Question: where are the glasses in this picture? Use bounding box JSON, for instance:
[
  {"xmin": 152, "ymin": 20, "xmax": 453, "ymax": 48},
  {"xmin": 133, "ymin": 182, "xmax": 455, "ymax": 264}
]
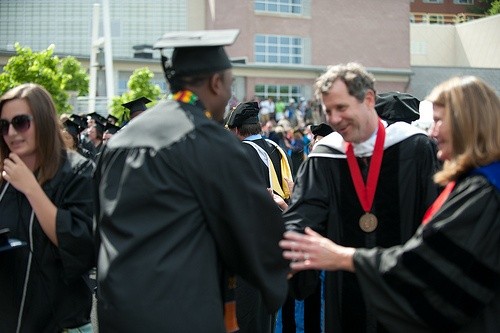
[{"xmin": 0, "ymin": 115, "xmax": 34, "ymax": 136}]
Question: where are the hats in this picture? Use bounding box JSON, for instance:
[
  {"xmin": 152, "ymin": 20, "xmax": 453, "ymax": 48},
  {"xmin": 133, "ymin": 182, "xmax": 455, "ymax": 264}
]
[
  {"xmin": 61, "ymin": 115, "xmax": 88, "ymax": 146},
  {"xmin": 373, "ymin": 91, "xmax": 420, "ymax": 123},
  {"xmin": 227, "ymin": 101, "xmax": 259, "ymax": 126},
  {"xmin": 153, "ymin": 29, "xmax": 241, "ymax": 71},
  {"xmin": 107, "ymin": 115, "xmax": 118, "ymax": 122},
  {"xmin": 95, "ymin": 120, "xmax": 105, "ymax": 132},
  {"xmin": 311, "ymin": 124, "xmax": 334, "ymax": 137},
  {"xmin": 122, "ymin": 97, "xmax": 152, "ymax": 122},
  {"xmin": 88, "ymin": 112, "xmax": 107, "ymax": 123},
  {"xmin": 105, "ymin": 123, "xmax": 120, "ymax": 134}
]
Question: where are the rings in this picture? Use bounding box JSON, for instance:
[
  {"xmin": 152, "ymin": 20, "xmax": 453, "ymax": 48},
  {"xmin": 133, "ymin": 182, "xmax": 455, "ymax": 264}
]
[
  {"xmin": 304, "ymin": 252, "xmax": 310, "ymax": 260},
  {"xmin": 304, "ymin": 260, "xmax": 311, "ymax": 267}
]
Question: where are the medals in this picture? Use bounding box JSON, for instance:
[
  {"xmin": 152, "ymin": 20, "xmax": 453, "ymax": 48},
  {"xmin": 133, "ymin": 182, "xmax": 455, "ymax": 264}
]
[{"xmin": 359, "ymin": 213, "xmax": 378, "ymax": 232}]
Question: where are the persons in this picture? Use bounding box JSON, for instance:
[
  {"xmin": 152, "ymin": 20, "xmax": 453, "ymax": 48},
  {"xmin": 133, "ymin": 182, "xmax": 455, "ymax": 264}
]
[
  {"xmin": 92, "ymin": 29, "xmax": 292, "ymax": 333},
  {"xmin": 0, "ymin": 83, "xmax": 97, "ymax": 333},
  {"xmin": 226, "ymin": 102, "xmax": 295, "ymax": 333},
  {"xmin": 60, "ymin": 91, "xmax": 420, "ymax": 182},
  {"xmin": 280, "ymin": 73, "xmax": 500, "ymax": 333},
  {"xmin": 282, "ymin": 61, "xmax": 444, "ymax": 333}
]
